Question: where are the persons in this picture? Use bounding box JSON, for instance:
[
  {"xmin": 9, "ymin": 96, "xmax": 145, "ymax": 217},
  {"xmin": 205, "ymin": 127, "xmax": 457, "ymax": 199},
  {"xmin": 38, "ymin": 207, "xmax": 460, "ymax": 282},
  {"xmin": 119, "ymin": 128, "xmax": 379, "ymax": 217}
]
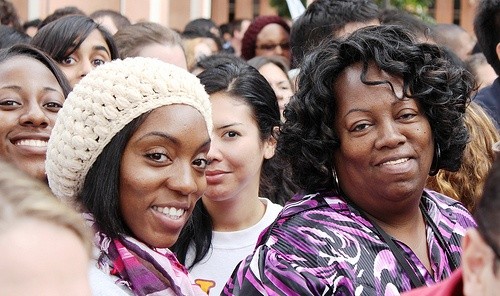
[
  {"xmin": 292, "ymin": 0, "xmax": 440, "ymax": 60},
  {"xmin": 180, "ymin": 16, "xmax": 300, "ymax": 128},
  {"xmin": 32, "ymin": 16, "xmax": 119, "ymax": 91},
  {"xmin": 221, "ymin": 25, "xmax": 479, "ymax": 296},
  {"xmin": 0, "ymin": 43, "xmax": 70, "ymax": 181},
  {"xmin": 0, "ymin": 0, "xmax": 131, "ymax": 42},
  {"xmin": 44, "ymin": 56, "xmax": 214, "ymax": 296},
  {"xmin": 111, "ymin": 21, "xmax": 188, "ymax": 72},
  {"xmin": 463, "ymin": 0, "xmax": 500, "ymax": 133},
  {"xmin": 178, "ymin": 62, "xmax": 284, "ymax": 296},
  {"xmin": 426, "ymin": 95, "xmax": 500, "ymax": 215},
  {"xmin": 462, "ymin": 153, "xmax": 500, "ymax": 296},
  {"xmin": 0, "ymin": 160, "xmax": 95, "ymax": 296}
]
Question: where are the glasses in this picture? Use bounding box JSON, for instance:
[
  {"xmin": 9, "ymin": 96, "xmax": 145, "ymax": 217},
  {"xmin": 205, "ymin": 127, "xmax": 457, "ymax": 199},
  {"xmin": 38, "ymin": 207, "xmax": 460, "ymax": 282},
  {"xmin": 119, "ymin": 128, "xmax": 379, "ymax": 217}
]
[{"xmin": 254, "ymin": 42, "xmax": 290, "ymax": 50}]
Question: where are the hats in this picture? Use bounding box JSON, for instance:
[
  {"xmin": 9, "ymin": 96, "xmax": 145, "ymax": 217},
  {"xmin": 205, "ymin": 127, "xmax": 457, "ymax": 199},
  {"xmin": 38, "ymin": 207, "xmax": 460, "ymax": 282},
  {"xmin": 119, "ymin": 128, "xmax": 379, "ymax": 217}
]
[
  {"xmin": 43, "ymin": 55, "xmax": 212, "ymax": 205},
  {"xmin": 183, "ymin": 18, "xmax": 223, "ymax": 49},
  {"xmin": 241, "ymin": 15, "xmax": 291, "ymax": 61}
]
